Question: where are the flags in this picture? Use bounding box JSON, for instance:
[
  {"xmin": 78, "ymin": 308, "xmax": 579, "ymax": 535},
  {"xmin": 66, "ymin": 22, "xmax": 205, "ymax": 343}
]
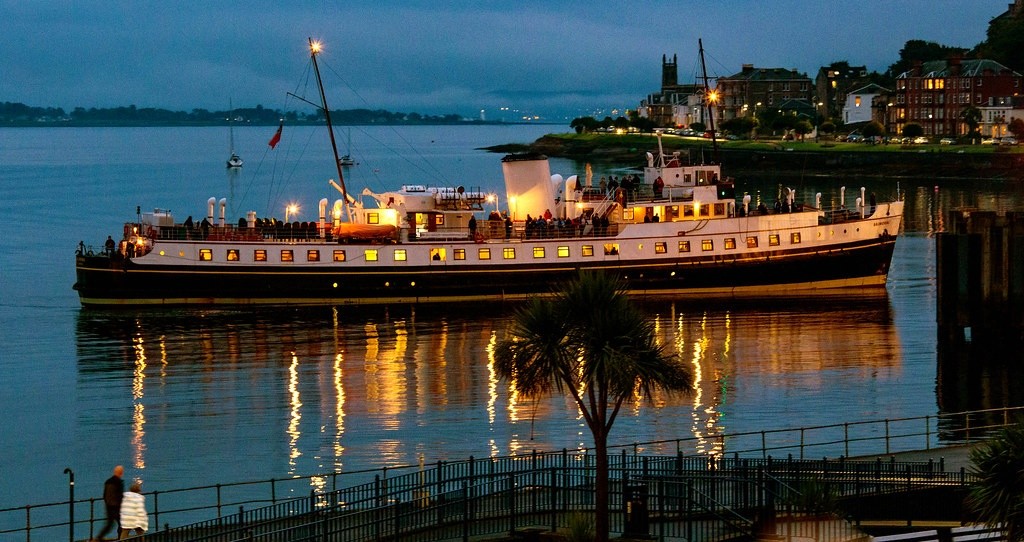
[{"xmin": 268, "ymin": 122, "xmax": 282, "ymax": 149}]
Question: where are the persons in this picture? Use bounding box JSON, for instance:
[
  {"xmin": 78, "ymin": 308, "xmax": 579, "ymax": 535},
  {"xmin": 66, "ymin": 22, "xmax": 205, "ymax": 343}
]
[
  {"xmin": 184, "ymin": 216, "xmax": 194, "ymax": 240},
  {"xmin": 469, "ymin": 174, "xmax": 797, "ymax": 239},
  {"xmin": 94, "ymin": 466, "xmax": 148, "ymax": 542},
  {"xmin": 201, "ymin": 218, "xmax": 214, "ymax": 241},
  {"xmin": 105, "ymin": 236, "xmax": 115, "ymax": 256}
]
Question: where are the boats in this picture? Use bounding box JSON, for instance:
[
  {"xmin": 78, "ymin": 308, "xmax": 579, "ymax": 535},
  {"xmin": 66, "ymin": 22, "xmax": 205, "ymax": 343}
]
[
  {"xmin": 67, "ymin": 35, "xmax": 906, "ymax": 313},
  {"xmin": 226, "ymin": 153, "xmax": 245, "ymax": 167},
  {"xmin": 337, "ymin": 155, "xmax": 355, "ymax": 167}
]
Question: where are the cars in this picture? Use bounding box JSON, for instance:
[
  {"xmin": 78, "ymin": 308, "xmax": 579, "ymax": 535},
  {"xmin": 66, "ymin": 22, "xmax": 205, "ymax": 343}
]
[
  {"xmin": 914, "ymin": 136, "xmax": 929, "ymax": 144},
  {"xmin": 835, "ymin": 130, "xmax": 915, "ymax": 144},
  {"xmin": 940, "ymin": 138, "xmax": 957, "ymax": 145},
  {"xmin": 653, "ymin": 127, "xmax": 741, "ymax": 141},
  {"xmin": 782, "ymin": 134, "xmax": 793, "ymax": 140},
  {"xmin": 982, "ymin": 138, "xmax": 1000, "ymax": 145},
  {"xmin": 1001, "ymin": 137, "xmax": 1018, "ymax": 145},
  {"xmin": 606, "ymin": 125, "xmax": 638, "ymax": 134}
]
